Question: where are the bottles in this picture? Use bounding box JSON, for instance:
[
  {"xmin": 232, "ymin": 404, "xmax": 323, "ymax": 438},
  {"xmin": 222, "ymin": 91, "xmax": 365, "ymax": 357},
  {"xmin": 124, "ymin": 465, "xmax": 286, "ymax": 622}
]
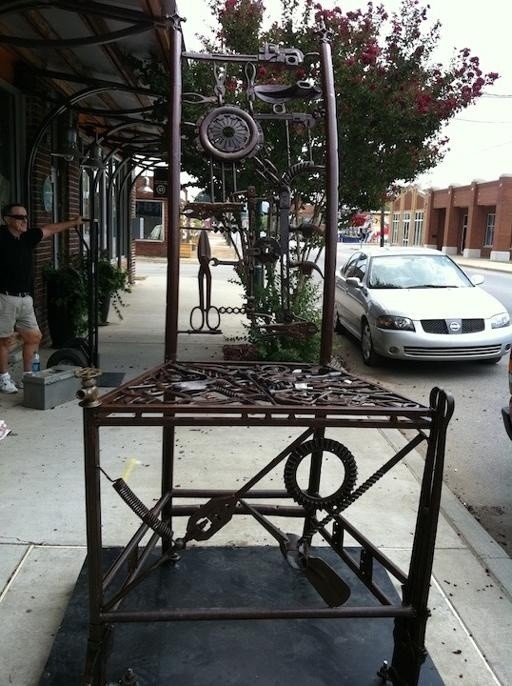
[{"xmin": 31, "ymin": 350, "xmax": 41, "ymax": 377}]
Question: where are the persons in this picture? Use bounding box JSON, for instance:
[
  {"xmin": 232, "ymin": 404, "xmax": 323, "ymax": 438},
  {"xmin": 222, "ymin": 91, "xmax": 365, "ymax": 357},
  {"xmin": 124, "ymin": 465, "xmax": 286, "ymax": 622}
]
[
  {"xmin": 356, "ymin": 228, "xmax": 365, "ymax": 248},
  {"xmin": 0, "ymin": 204, "xmax": 86, "ymax": 393}
]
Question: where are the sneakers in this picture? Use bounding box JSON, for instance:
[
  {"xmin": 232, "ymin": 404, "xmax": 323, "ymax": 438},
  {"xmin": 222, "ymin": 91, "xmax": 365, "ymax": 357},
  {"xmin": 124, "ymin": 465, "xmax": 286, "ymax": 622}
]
[{"xmin": 0, "ymin": 376, "xmax": 18, "ymax": 394}]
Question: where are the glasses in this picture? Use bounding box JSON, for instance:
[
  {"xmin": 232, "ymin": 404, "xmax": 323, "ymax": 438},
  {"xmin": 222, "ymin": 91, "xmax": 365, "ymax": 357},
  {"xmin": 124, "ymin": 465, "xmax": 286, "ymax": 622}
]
[{"xmin": 7, "ymin": 214, "xmax": 27, "ymax": 219}]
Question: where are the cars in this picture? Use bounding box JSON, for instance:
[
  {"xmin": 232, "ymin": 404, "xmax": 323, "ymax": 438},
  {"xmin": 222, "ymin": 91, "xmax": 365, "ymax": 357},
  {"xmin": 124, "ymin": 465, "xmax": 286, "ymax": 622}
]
[{"xmin": 334, "ymin": 246, "xmax": 511, "ymax": 367}]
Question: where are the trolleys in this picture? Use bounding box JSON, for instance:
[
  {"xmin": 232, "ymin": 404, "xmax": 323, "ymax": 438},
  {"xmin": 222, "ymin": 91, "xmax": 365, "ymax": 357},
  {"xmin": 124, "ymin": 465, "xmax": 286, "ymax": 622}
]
[{"xmin": 46, "ymin": 219, "xmax": 126, "ymax": 387}]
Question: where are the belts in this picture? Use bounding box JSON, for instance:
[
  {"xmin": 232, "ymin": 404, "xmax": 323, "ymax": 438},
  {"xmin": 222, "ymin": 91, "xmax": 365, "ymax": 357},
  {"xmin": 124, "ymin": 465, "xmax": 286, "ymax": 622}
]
[{"xmin": 1, "ymin": 290, "xmax": 30, "ymax": 297}]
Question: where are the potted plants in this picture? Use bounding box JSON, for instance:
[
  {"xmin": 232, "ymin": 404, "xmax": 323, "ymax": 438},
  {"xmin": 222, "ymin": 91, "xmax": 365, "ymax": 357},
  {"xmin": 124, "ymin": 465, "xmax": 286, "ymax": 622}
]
[{"xmin": 41, "ymin": 246, "xmax": 137, "ymax": 349}]
